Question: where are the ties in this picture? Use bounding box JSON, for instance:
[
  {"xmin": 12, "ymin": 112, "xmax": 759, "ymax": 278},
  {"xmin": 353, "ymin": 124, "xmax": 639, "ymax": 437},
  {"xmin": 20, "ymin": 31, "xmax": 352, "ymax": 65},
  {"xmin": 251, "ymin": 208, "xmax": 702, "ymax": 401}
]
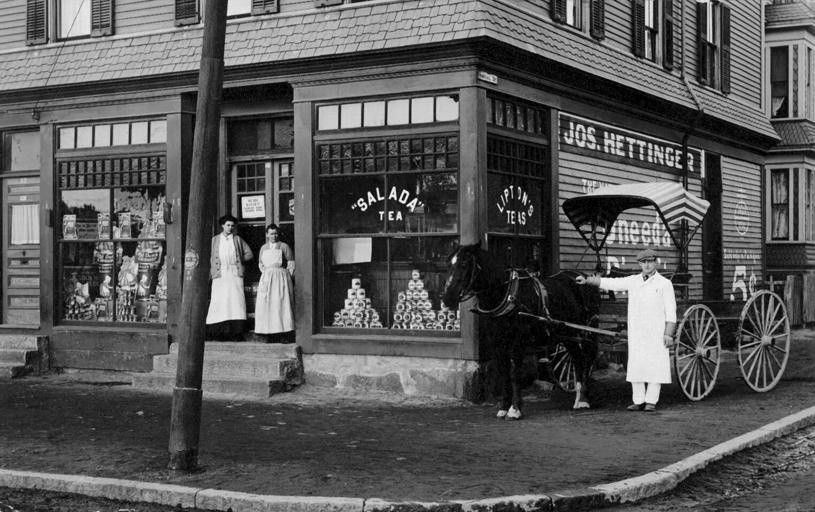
[{"xmin": 643, "ymin": 274, "xmax": 649, "ymax": 281}]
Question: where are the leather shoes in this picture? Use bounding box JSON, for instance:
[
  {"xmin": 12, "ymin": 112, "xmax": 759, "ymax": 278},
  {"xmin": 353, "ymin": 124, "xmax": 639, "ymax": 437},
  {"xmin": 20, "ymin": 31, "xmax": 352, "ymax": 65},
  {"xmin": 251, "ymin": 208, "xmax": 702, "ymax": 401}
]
[
  {"xmin": 627, "ymin": 404, "xmax": 644, "ymax": 411},
  {"xmin": 644, "ymin": 403, "xmax": 656, "ymax": 411}
]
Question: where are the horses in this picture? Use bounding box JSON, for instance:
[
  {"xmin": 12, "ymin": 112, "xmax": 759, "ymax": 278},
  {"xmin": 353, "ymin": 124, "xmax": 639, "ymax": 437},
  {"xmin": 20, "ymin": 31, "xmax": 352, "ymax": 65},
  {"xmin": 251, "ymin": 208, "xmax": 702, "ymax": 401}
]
[{"xmin": 438, "ymin": 238, "xmax": 602, "ymax": 422}]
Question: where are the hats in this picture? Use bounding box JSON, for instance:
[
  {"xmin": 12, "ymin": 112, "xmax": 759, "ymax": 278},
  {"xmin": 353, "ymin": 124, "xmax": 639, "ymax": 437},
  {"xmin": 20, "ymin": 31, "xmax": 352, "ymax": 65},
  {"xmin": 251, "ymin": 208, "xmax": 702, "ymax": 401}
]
[{"xmin": 636, "ymin": 250, "xmax": 657, "ymax": 263}]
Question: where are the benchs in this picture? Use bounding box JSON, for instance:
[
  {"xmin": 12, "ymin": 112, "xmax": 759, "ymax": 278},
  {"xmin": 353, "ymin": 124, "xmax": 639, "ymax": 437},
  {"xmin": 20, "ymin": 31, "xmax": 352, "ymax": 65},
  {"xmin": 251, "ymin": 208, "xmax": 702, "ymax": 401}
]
[{"xmin": 603, "ymin": 267, "xmax": 693, "ymax": 299}]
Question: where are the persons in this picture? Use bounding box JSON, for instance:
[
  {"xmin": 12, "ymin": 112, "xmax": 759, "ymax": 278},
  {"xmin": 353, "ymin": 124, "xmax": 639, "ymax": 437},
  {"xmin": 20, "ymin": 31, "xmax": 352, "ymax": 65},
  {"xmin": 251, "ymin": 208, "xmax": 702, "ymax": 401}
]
[
  {"xmin": 206, "ymin": 212, "xmax": 254, "ymax": 343},
  {"xmin": 574, "ymin": 248, "xmax": 677, "ymax": 412},
  {"xmin": 255, "ymin": 223, "xmax": 295, "ymax": 344}
]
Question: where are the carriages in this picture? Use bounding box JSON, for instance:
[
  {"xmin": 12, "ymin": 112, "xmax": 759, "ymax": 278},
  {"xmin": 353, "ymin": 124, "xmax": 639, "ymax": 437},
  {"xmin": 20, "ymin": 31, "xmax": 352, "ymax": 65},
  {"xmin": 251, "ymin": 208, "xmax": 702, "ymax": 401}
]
[{"xmin": 439, "ymin": 181, "xmax": 790, "ymax": 420}]
